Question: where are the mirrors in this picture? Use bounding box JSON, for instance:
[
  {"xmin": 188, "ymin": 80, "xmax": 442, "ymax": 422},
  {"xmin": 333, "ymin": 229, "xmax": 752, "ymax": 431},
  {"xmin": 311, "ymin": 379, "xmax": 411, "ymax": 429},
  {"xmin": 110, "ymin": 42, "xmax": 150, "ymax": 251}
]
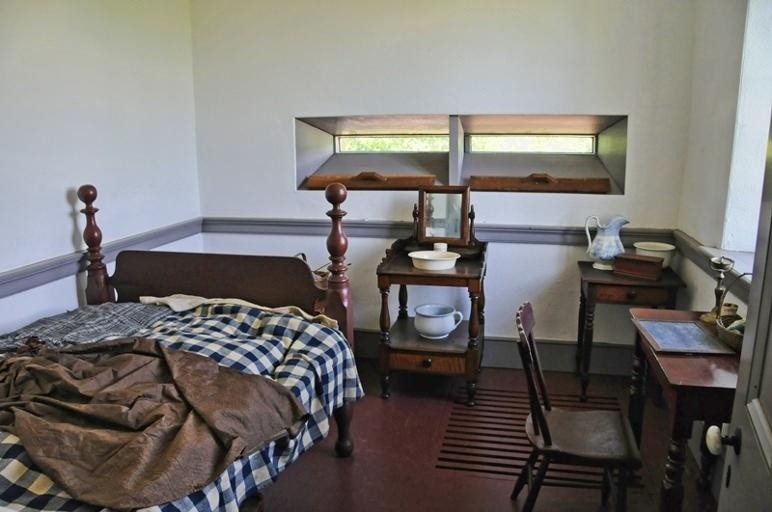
[{"xmin": 412, "ymin": 185, "xmax": 484, "ymax": 248}]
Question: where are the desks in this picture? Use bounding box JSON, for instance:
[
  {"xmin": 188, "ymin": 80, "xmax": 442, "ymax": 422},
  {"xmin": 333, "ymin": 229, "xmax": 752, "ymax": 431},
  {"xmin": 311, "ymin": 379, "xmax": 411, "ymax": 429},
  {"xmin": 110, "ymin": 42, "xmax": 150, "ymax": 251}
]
[
  {"xmin": 628, "ymin": 310, "xmax": 741, "ymax": 512},
  {"xmin": 577, "ymin": 262, "xmax": 685, "ymax": 404},
  {"xmin": 377, "ymin": 253, "xmax": 487, "ymax": 407}
]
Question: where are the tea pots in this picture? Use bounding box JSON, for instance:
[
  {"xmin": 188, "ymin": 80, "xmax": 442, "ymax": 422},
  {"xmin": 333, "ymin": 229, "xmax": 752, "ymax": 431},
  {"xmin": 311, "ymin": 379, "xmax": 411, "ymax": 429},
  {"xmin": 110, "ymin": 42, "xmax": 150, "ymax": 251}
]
[{"xmin": 585, "ymin": 217, "xmax": 630, "ymax": 271}]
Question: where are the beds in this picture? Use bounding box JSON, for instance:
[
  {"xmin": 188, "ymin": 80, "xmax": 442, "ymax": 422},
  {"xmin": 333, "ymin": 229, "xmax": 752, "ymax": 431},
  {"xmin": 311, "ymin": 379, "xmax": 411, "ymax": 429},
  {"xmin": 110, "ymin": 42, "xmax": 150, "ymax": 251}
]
[{"xmin": 0, "ymin": 183, "xmax": 366, "ymax": 511}]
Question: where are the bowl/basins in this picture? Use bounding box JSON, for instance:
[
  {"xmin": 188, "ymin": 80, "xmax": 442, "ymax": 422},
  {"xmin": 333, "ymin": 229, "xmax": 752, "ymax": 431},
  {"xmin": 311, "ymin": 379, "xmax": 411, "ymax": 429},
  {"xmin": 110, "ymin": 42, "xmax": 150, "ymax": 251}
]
[
  {"xmin": 634, "ymin": 239, "xmax": 677, "ymax": 268},
  {"xmin": 406, "ymin": 250, "xmax": 460, "ymax": 270}
]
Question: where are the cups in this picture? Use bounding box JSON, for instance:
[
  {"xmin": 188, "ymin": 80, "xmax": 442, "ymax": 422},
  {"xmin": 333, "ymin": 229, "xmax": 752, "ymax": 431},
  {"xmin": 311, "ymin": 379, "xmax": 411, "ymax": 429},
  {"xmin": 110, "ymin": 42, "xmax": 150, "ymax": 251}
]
[{"xmin": 413, "ymin": 303, "xmax": 463, "ymax": 341}]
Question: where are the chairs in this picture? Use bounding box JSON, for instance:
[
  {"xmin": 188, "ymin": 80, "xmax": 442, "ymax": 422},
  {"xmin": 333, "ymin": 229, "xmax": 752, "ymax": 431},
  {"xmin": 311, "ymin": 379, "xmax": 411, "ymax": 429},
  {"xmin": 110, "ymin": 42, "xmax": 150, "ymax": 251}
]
[{"xmin": 510, "ymin": 303, "xmax": 643, "ymax": 512}]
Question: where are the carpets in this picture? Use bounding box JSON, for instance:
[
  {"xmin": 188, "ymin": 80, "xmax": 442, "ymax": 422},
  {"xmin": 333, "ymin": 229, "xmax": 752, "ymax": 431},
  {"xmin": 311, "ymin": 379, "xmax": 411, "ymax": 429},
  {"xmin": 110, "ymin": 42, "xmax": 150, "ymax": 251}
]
[{"xmin": 434, "ymin": 383, "xmax": 652, "ymax": 490}]
